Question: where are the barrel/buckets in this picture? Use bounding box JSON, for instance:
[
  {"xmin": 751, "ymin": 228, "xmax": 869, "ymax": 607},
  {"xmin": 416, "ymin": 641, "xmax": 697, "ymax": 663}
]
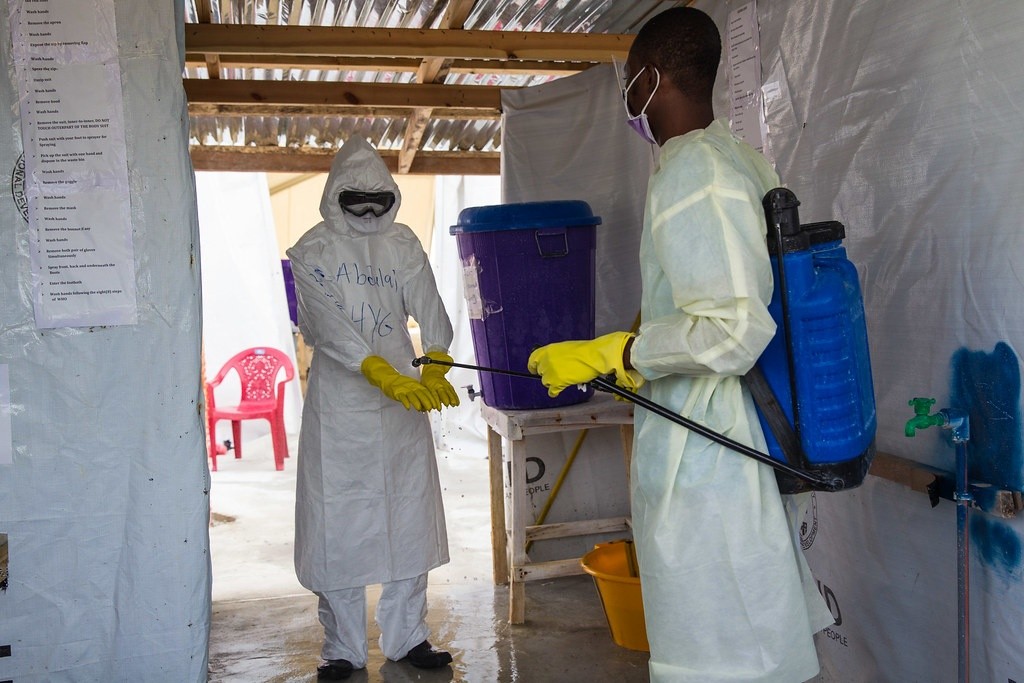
[
  {"xmin": 580, "ymin": 540, "xmax": 651, "ymax": 651},
  {"xmin": 750, "ymin": 187, "xmax": 878, "ymax": 493},
  {"xmin": 450, "ymin": 199, "xmax": 602, "ymax": 410}
]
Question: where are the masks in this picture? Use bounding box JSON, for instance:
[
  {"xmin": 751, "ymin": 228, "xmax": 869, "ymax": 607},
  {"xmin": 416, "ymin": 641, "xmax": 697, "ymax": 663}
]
[
  {"xmin": 623, "ymin": 66, "xmax": 660, "ymax": 144},
  {"xmin": 342, "ymin": 203, "xmax": 386, "ymax": 233}
]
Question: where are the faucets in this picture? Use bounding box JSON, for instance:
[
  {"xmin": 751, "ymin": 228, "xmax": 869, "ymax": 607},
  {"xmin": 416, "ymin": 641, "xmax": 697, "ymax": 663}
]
[{"xmin": 902, "ymin": 396, "xmax": 945, "ymax": 439}]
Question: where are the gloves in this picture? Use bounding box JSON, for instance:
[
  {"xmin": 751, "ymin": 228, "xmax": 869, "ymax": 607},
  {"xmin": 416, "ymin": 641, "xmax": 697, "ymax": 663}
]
[
  {"xmin": 528, "ymin": 331, "xmax": 645, "ymax": 398},
  {"xmin": 613, "ymin": 380, "xmax": 641, "ymax": 405},
  {"xmin": 361, "ymin": 356, "xmax": 437, "ymax": 412},
  {"xmin": 421, "ymin": 351, "xmax": 460, "ymax": 407}
]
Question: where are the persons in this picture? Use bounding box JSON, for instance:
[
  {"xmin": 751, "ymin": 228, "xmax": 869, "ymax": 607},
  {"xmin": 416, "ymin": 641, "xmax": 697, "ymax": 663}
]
[
  {"xmin": 287, "ymin": 133, "xmax": 462, "ymax": 683},
  {"xmin": 526, "ymin": 6, "xmax": 835, "ymax": 683}
]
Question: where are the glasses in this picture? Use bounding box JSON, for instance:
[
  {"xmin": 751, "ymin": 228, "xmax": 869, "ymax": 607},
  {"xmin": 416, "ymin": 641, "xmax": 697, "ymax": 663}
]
[{"xmin": 338, "ymin": 191, "xmax": 396, "ymax": 217}]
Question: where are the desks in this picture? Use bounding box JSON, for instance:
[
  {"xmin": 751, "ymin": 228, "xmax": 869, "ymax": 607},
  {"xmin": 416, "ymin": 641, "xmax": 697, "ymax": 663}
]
[{"xmin": 481, "ymin": 390, "xmax": 635, "ymax": 626}]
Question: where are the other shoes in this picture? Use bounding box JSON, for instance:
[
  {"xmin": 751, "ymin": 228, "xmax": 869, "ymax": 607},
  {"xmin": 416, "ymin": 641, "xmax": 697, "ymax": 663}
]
[
  {"xmin": 316, "ymin": 659, "xmax": 352, "ymax": 680},
  {"xmin": 387, "ymin": 639, "xmax": 453, "ymax": 668}
]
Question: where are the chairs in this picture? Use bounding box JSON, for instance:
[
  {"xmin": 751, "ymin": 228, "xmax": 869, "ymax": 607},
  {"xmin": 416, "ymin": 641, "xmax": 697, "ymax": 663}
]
[{"xmin": 205, "ymin": 347, "xmax": 295, "ymax": 470}]
